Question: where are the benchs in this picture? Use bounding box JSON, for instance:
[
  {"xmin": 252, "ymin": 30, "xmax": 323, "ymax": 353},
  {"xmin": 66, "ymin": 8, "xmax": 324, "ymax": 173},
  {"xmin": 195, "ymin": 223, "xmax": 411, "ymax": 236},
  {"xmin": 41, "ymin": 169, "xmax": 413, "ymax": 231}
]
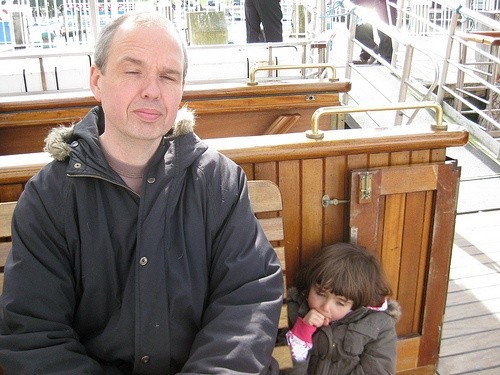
[{"xmin": 0, "ymin": 180, "xmax": 294, "ymax": 375}]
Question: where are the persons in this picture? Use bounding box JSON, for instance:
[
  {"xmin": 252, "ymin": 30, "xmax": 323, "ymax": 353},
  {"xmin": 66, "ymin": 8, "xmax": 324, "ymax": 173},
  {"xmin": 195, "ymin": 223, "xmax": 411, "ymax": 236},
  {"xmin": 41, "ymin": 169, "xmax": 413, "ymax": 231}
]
[
  {"xmin": 271, "ymin": 240, "xmax": 402, "ymax": 375},
  {"xmin": 244, "ymin": 0, "xmax": 283, "ymax": 42},
  {"xmin": 345, "ymin": 11, "xmax": 393, "ymax": 65},
  {"xmin": 0, "ymin": 10, "xmax": 285, "ymax": 375}
]
